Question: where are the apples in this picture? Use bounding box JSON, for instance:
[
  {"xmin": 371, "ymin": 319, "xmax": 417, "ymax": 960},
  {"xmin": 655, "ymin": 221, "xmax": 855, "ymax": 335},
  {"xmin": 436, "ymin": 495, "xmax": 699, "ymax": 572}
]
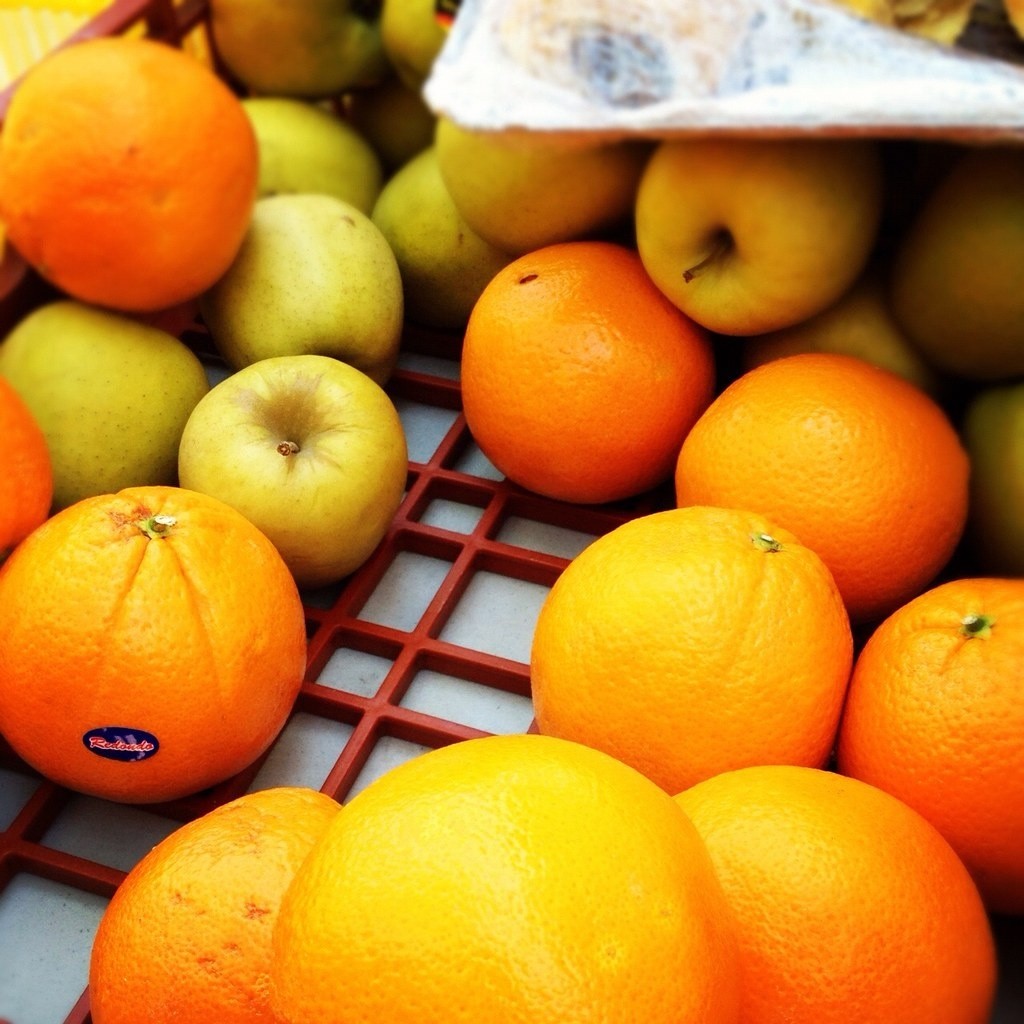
[{"xmin": 0, "ymin": 0, "xmax": 1024, "ymax": 594}]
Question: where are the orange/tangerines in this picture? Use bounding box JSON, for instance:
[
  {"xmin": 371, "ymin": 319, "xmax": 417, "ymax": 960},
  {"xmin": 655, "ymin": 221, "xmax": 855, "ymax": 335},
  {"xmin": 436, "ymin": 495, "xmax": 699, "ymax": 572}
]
[{"xmin": 0, "ymin": 36, "xmax": 1024, "ymax": 1024}]
